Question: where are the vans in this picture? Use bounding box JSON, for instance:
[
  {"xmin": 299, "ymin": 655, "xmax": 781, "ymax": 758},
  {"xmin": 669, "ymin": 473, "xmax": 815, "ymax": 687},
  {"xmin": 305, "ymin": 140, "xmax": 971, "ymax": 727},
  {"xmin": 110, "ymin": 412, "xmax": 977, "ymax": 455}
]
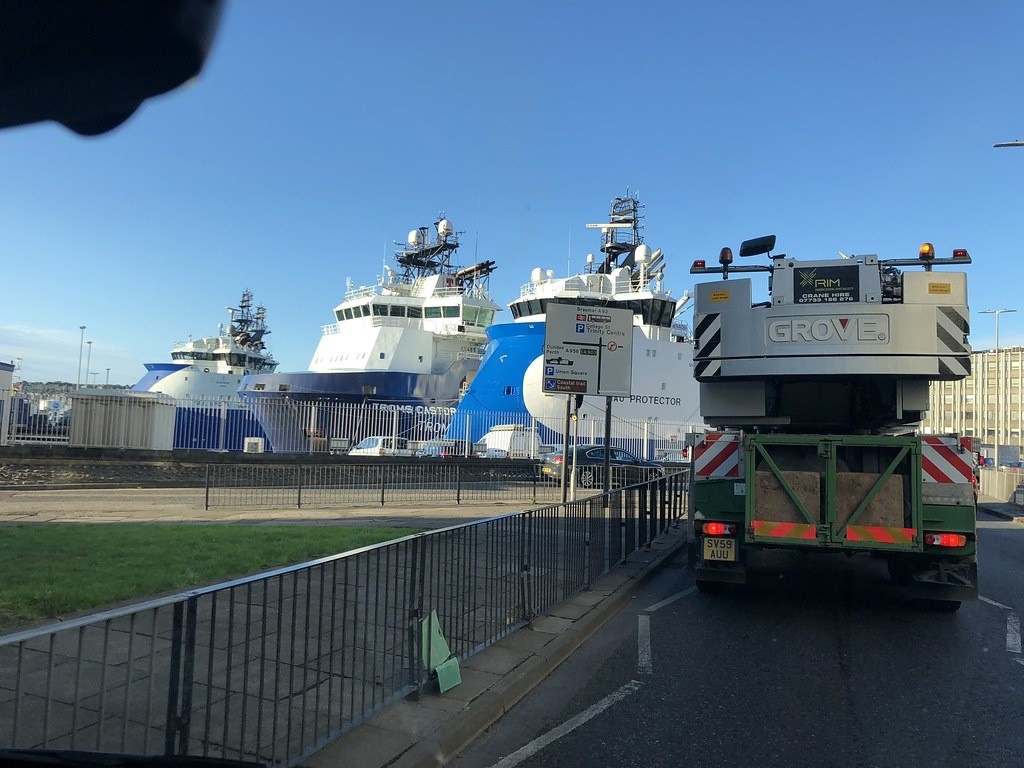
[
  {"xmin": 347, "ymin": 435, "xmax": 410, "ymax": 456},
  {"xmin": 475, "ymin": 425, "xmax": 545, "ymax": 460}
]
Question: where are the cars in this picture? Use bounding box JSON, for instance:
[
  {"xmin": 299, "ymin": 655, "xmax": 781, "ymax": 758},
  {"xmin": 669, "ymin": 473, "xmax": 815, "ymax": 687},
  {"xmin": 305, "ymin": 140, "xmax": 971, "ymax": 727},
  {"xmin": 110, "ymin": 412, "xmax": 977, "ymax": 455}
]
[
  {"xmin": 544, "ymin": 445, "xmax": 665, "ymax": 489},
  {"xmin": 979, "ymin": 455, "xmax": 1024, "ymax": 472}
]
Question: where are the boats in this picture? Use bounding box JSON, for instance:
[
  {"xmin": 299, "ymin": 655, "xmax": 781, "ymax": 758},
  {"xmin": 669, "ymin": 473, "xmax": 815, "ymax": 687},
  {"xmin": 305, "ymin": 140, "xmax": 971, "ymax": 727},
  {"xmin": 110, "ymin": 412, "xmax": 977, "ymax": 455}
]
[
  {"xmin": 124, "ymin": 287, "xmax": 277, "ymax": 452},
  {"xmin": 425, "ymin": 182, "xmax": 716, "ymax": 464},
  {"xmin": 236, "ymin": 207, "xmax": 505, "ymax": 453}
]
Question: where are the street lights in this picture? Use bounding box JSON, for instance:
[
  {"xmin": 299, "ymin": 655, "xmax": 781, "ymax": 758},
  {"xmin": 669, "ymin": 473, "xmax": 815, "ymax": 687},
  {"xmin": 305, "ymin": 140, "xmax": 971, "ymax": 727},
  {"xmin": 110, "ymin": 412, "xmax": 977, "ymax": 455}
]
[
  {"xmin": 106, "ymin": 368, "xmax": 112, "ymax": 385},
  {"xmin": 91, "ymin": 372, "xmax": 99, "ymax": 386},
  {"xmin": 979, "ymin": 308, "xmax": 1018, "ymax": 470},
  {"xmin": 85, "ymin": 340, "xmax": 93, "ymax": 385},
  {"xmin": 76, "ymin": 326, "xmax": 87, "ymax": 389}
]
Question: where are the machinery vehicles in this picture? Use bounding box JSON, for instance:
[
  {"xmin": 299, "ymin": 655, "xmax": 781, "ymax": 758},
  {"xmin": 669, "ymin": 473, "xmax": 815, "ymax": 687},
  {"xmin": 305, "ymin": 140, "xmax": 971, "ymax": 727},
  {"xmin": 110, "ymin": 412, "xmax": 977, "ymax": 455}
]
[{"xmin": 690, "ymin": 235, "xmax": 981, "ymax": 608}]
